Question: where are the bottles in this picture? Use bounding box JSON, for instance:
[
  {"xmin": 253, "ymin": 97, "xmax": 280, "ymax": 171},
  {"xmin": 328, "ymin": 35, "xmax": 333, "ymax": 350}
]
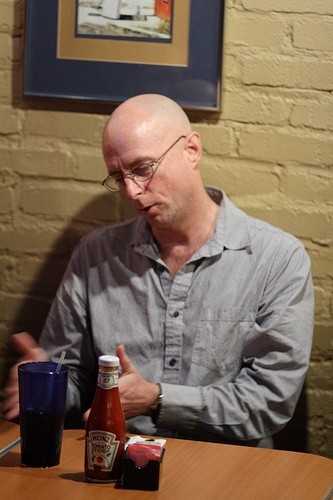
[{"xmin": 83, "ymin": 353, "xmax": 125, "ymax": 485}]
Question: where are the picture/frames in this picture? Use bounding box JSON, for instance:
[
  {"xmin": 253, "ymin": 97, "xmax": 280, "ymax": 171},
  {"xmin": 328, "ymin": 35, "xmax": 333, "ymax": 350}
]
[{"xmin": 23, "ymin": 0, "xmax": 225, "ymax": 114}]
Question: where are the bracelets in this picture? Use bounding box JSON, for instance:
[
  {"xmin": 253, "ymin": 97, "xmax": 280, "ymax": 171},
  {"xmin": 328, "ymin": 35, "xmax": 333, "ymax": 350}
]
[{"xmin": 150, "ymin": 394, "xmax": 163, "ymax": 425}]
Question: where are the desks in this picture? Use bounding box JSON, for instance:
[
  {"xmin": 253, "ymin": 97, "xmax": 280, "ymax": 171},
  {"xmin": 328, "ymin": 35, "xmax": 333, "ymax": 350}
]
[{"xmin": 0, "ymin": 421, "xmax": 333, "ymax": 500}]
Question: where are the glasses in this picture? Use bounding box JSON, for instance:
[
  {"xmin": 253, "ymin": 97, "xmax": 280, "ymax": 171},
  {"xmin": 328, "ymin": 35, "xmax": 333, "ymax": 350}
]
[{"xmin": 102, "ymin": 136, "xmax": 187, "ymax": 193}]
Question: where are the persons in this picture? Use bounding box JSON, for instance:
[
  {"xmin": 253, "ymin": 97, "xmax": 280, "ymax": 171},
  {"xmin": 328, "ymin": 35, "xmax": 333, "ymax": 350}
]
[{"xmin": 3, "ymin": 94, "xmax": 315, "ymax": 448}]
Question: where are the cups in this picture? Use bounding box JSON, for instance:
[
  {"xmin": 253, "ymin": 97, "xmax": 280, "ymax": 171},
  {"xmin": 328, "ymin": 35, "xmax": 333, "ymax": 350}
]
[{"xmin": 17, "ymin": 358, "xmax": 69, "ymax": 469}]
